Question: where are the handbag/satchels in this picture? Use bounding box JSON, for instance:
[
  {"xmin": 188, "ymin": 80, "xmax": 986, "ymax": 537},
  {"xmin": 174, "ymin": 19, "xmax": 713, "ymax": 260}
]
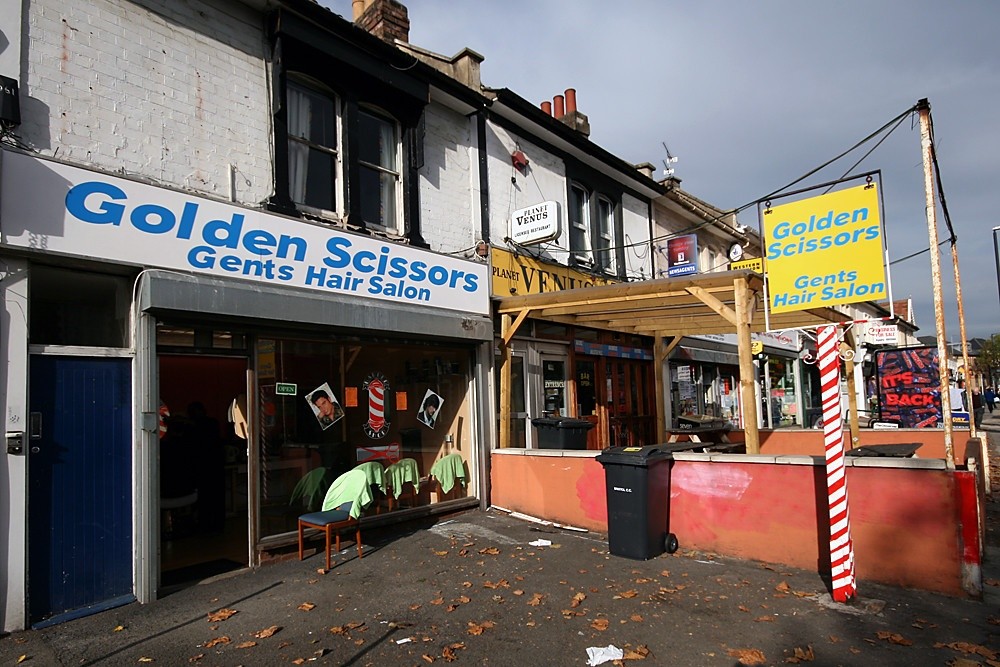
[{"xmin": 993, "ymin": 395, "xmax": 1000, "ymax": 402}]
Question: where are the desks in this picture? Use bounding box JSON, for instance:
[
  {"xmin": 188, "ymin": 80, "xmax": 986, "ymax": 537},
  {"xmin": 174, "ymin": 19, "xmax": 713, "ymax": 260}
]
[{"xmin": 654, "ymin": 427, "xmax": 731, "ymax": 454}]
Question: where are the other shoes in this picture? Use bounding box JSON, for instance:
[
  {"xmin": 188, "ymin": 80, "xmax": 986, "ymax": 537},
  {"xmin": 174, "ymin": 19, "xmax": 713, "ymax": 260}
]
[{"xmin": 977, "ymin": 426, "xmax": 981, "ymax": 430}]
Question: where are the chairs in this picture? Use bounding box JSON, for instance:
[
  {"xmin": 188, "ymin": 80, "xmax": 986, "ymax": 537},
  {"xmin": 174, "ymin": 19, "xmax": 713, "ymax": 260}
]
[
  {"xmin": 298, "ymin": 455, "xmax": 462, "ymax": 571},
  {"xmin": 289, "ymin": 467, "xmax": 326, "ymax": 513}
]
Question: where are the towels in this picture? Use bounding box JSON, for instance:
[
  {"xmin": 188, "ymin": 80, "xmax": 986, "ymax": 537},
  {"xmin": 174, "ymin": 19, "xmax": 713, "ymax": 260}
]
[
  {"xmin": 384, "ymin": 458, "xmax": 421, "ymax": 500},
  {"xmin": 431, "ymin": 453, "xmax": 466, "ymax": 494},
  {"xmin": 322, "ymin": 461, "xmax": 387, "ymax": 521},
  {"xmin": 289, "ymin": 466, "xmax": 334, "ymax": 513}
]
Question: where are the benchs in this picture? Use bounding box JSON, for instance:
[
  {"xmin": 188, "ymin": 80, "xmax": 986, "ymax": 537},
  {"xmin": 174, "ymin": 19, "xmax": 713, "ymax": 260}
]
[{"xmin": 711, "ymin": 442, "xmax": 745, "ymax": 453}]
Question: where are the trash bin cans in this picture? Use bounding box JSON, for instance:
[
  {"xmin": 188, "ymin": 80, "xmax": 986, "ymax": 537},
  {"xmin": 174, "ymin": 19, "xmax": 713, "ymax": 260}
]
[
  {"xmin": 531, "ymin": 417, "xmax": 594, "ymax": 450},
  {"xmin": 595, "ymin": 446, "xmax": 677, "ymax": 560}
]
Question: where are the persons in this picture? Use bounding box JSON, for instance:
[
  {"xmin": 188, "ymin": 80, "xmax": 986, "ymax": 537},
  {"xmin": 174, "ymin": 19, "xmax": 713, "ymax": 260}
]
[
  {"xmin": 310, "ymin": 388, "xmax": 343, "ymax": 430},
  {"xmin": 419, "ymin": 394, "xmax": 440, "ymax": 428},
  {"xmin": 951, "ymin": 380, "xmax": 1000, "ymax": 430}
]
